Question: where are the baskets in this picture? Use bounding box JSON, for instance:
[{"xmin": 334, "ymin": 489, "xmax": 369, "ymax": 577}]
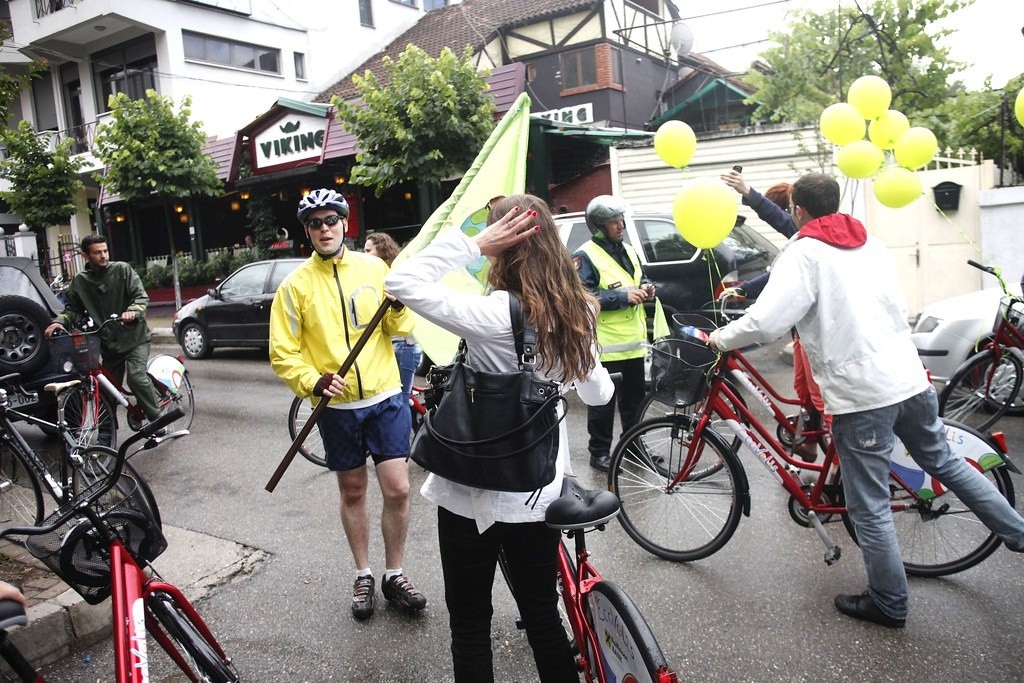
[
  {"xmin": 671, "ymin": 313, "xmax": 719, "ymax": 343},
  {"xmin": 24, "ymin": 471, "xmax": 168, "ymax": 606},
  {"xmin": 647, "ymin": 339, "xmax": 720, "ymax": 407},
  {"xmin": 46, "ymin": 333, "xmax": 101, "ymax": 372},
  {"xmin": 991, "ymin": 301, "xmax": 1024, "ymax": 343}
]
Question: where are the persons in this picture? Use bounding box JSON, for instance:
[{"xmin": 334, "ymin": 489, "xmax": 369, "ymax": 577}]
[
  {"xmin": 710, "ymin": 172, "xmax": 1024, "ymax": 628},
  {"xmin": 365, "ymin": 233, "xmax": 422, "ymax": 432},
  {"xmin": 383, "ymin": 194, "xmax": 615, "ymax": 683},
  {"xmin": 720, "ymin": 169, "xmax": 833, "ymax": 463},
  {"xmin": 45, "ymin": 234, "xmax": 166, "ymax": 447},
  {"xmin": 569, "ymin": 194, "xmax": 664, "ymax": 472},
  {"xmin": 270, "ymin": 188, "xmax": 428, "ymax": 619}
]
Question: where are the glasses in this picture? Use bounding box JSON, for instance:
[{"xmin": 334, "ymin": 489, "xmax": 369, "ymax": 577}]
[{"xmin": 305, "ymin": 214, "xmax": 345, "ymax": 227}]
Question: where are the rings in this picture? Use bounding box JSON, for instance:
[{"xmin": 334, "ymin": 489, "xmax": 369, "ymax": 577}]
[{"xmin": 508, "ymin": 222, "xmax": 512, "ymax": 227}]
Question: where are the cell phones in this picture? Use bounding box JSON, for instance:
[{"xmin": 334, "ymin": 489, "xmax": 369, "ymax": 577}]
[{"xmin": 733, "ymin": 165, "xmax": 742, "ymax": 173}]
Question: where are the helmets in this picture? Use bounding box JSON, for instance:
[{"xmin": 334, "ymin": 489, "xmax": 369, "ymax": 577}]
[
  {"xmin": 296, "ymin": 187, "xmax": 350, "ymax": 223},
  {"xmin": 584, "ymin": 195, "xmax": 627, "ymax": 243}
]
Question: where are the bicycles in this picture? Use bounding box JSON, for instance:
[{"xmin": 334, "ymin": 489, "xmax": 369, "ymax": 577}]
[
  {"xmin": 608, "ymin": 271, "xmax": 1022, "ymax": 577},
  {"xmin": 0, "ymin": 369, "xmax": 242, "ymax": 683},
  {"xmin": 937, "ymin": 259, "xmax": 1024, "ymax": 432},
  {"xmin": 44, "ymin": 312, "xmax": 195, "ymax": 478},
  {"xmin": 287, "ymin": 312, "xmax": 681, "ymax": 682}
]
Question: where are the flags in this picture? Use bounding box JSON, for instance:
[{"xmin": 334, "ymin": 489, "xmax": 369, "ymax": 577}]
[{"xmin": 393, "ymin": 93, "xmax": 531, "ymax": 366}]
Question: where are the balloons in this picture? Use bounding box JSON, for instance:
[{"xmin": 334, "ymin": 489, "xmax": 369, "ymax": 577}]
[
  {"xmin": 672, "ymin": 176, "xmax": 737, "ymax": 249},
  {"xmin": 654, "ymin": 120, "xmax": 697, "ymax": 169},
  {"xmin": 820, "ymin": 75, "xmax": 937, "ymax": 207}
]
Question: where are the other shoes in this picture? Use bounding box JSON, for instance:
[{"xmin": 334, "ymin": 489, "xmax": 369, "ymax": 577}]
[
  {"xmin": 627, "ymin": 438, "xmax": 664, "ymax": 462},
  {"xmin": 835, "ymin": 590, "xmax": 908, "ymax": 628},
  {"xmin": 589, "ymin": 454, "xmax": 623, "ymax": 475},
  {"xmin": 380, "ymin": 571, "xmax": 428, "ymax": 610},
  {"xmin": 153, "ymin": 424, "xmax": 169, "ymax": 438},
  {"xmin": 351, "ymin": 575, "xmax": 376, "ymax": 619}
]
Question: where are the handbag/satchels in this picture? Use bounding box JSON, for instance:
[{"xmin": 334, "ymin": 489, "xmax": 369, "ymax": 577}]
[{"xmin": 403, "ymin": 290, "xmax": 570, "ymax": 512}]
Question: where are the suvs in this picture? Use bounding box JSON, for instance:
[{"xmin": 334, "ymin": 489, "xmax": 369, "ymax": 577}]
[
  {"xmin": 546, "ymin": 211, "xmax": 781, "ymax": 363},
  {"xmin": 0, "ymin": 256, "xmax": 85, "ymax": 439}
]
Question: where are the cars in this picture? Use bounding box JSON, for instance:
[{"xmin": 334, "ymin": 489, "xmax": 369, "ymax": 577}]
[
  {"xmin": 907, "ymin": 280, "xmax": 1024, "ymax": 416},
  {"xmin": 172, "ymin": 258, "xmax": 310, "ymax": 360}
]
